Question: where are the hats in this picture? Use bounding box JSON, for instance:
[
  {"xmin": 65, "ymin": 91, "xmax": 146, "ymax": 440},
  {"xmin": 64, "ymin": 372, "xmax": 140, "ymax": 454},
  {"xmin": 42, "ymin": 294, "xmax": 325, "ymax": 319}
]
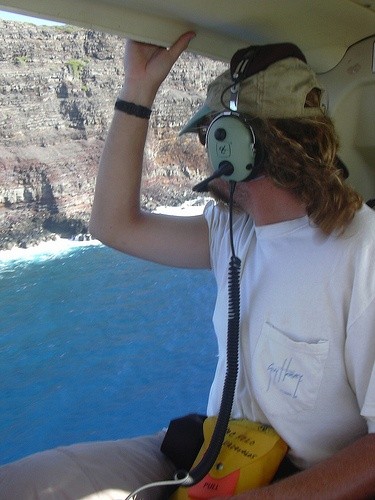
[{"xmin": 178, "ymin": 57, "xmax": 326, "ymax": 136}]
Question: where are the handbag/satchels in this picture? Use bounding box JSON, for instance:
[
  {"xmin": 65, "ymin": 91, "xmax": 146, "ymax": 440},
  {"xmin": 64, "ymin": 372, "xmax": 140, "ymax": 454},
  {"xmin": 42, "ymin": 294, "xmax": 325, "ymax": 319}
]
[{"xmin": 174, "ymin": 417, "xmax": 289, "ymax": 500}]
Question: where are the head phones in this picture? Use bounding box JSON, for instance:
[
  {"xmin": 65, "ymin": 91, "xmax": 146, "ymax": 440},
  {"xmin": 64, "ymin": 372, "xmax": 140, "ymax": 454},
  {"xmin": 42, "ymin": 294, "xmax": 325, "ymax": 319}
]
[{"xmin": 204, "ymin": 44, "xmax": 262, "ymax": 182}]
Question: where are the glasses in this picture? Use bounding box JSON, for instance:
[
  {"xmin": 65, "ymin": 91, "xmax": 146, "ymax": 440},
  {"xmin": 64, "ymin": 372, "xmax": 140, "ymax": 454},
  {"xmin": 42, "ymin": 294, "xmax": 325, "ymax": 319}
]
[{"xmin": 199, "ymin": 127, "xmax": 207, "ymax": 146}]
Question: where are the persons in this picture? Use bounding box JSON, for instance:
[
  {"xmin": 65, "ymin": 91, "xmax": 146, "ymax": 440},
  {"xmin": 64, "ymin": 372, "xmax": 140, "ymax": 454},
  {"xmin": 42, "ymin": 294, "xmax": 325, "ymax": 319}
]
[{"xmin": 0, "ymin": 29, "xmax": 375, "ymax": 500}]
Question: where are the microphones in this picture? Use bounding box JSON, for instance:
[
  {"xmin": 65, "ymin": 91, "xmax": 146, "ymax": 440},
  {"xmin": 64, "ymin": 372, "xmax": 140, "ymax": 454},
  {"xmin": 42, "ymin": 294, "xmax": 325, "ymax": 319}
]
[{"xmin": 191, "ymin": 164, "xmax": 231, "ymax": 193}]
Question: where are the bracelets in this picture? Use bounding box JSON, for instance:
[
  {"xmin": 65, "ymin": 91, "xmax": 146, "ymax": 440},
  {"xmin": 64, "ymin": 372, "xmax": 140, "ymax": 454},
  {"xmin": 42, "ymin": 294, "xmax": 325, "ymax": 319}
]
[{"xmin": 114, "ymin": 98, "xmax": 152, "ymax": 121}]
